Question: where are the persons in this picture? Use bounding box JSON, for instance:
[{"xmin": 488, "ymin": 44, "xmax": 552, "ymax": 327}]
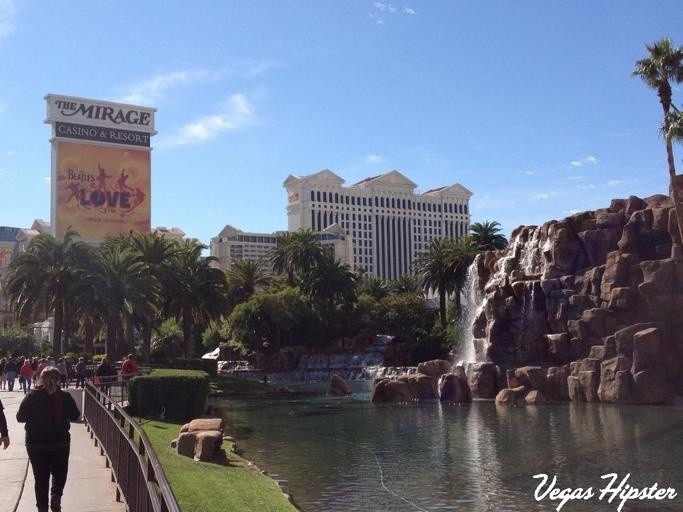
[
  {"xmin": 0, "ymin": 353, "xmax": 139, "ymax": 394},
  {"xmin": 16, "ymin": 365, "xmax": 80, "ymax": 512},
  {"xmin": 0, "ymin": 398, "xmax": 10, "ymax": 450}
]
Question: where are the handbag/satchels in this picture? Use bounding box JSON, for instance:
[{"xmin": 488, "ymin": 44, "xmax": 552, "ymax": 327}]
[
  {"xmin": 19, "ymin": 378, "xmax": 23, "ymax": 383},
  {"xmin": 50, "ymin": 433, "xmax": 70, "ymax": 465}
]
